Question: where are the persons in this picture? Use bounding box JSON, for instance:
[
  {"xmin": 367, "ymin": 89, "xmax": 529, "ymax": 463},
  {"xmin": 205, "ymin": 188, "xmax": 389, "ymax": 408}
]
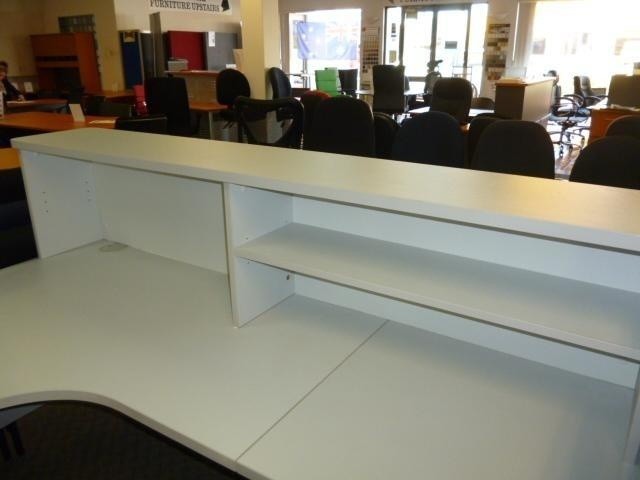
[{"xmin": 0, "ymin": 60, "xmax": 26, "ymax": 102}]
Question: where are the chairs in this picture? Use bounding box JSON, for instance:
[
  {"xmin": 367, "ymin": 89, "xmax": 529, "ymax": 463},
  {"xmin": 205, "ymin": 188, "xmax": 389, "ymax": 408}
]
[{"xmin": 86, "ymin": 63, "xmax": 639, "ymax": 189}]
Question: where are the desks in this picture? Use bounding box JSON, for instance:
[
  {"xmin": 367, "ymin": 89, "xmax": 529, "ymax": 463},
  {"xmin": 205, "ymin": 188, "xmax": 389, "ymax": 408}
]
[
  {"xmin": 0, "ymin": 126, "xmax": 639, "ymax": 480},
  {"xmin": 0, "ymin": 106, "xmax": 120, "ymax": 133}
]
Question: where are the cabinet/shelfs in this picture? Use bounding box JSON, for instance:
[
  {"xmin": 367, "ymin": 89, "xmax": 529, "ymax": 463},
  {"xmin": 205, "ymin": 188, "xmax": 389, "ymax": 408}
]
[{"xmin": 27, "ymin": 28, "xmax": 103, "ymax": 97}]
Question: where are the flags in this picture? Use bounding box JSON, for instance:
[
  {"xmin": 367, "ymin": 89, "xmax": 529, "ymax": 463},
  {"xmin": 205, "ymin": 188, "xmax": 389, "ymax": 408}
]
[{"xmin": 297, "ymin": 21, "xmax": 357, "ymax": 60}]
